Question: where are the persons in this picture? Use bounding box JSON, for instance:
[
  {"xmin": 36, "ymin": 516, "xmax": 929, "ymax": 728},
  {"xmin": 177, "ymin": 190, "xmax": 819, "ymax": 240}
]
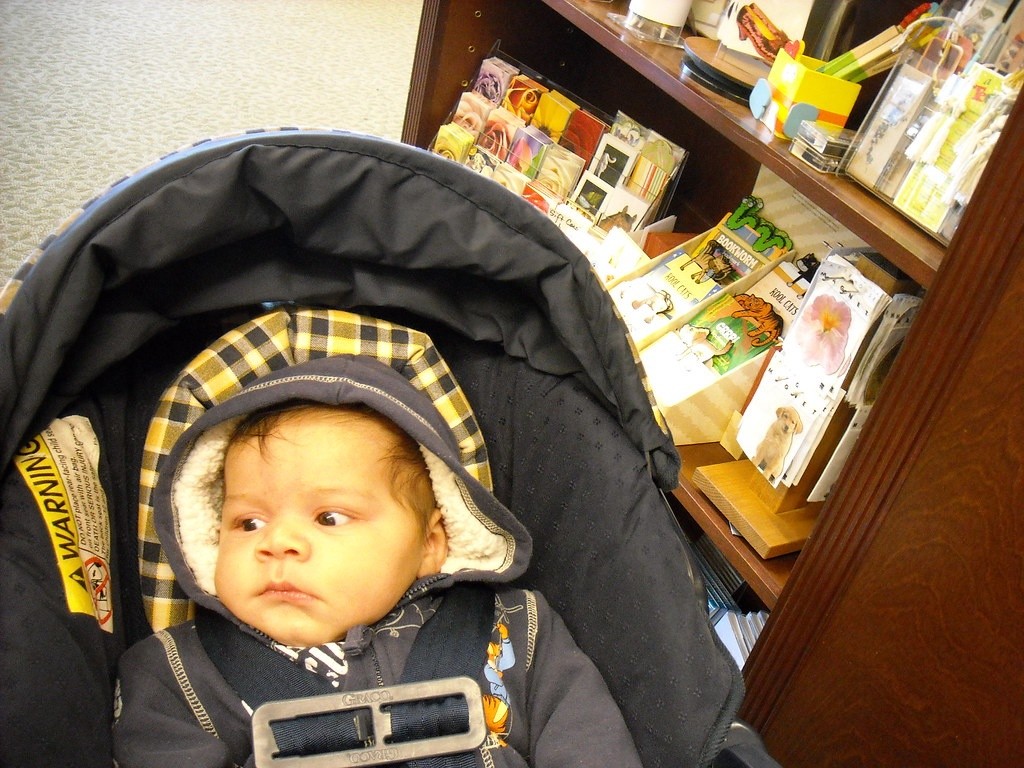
[{"xmin": 112, "ymin": 354, "xmax": 643, "ymax": 768}]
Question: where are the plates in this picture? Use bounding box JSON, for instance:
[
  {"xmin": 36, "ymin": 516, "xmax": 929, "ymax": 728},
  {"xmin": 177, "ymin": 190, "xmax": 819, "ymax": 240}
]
[{"xmin": 683, "ymin": 36, "xmax": 772, "ymax": 94}]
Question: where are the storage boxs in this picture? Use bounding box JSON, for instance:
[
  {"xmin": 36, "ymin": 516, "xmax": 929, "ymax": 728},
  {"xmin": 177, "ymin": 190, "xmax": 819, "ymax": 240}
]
[{"xmin": 749, "ymin": 48, "xmax": 862, "ymax": 145}]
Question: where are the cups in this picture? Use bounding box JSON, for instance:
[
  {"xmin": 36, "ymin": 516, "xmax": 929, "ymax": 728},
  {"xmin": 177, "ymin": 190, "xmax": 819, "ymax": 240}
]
[{"xmin": 625, "ymin": 0, "xmax": 691, "ymax": 46}]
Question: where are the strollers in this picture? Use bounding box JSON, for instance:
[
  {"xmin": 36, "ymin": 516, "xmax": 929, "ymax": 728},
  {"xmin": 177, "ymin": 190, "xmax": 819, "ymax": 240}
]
[{"xmin": 0, "ymin": 130, "xmax": 790, "ymax": 767}]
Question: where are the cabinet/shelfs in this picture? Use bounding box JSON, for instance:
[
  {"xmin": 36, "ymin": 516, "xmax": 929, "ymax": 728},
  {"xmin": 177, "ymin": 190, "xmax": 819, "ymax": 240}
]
[{"xmin": 400, "ymin": 1, "xmax": 1024, "ymax": 768}]
